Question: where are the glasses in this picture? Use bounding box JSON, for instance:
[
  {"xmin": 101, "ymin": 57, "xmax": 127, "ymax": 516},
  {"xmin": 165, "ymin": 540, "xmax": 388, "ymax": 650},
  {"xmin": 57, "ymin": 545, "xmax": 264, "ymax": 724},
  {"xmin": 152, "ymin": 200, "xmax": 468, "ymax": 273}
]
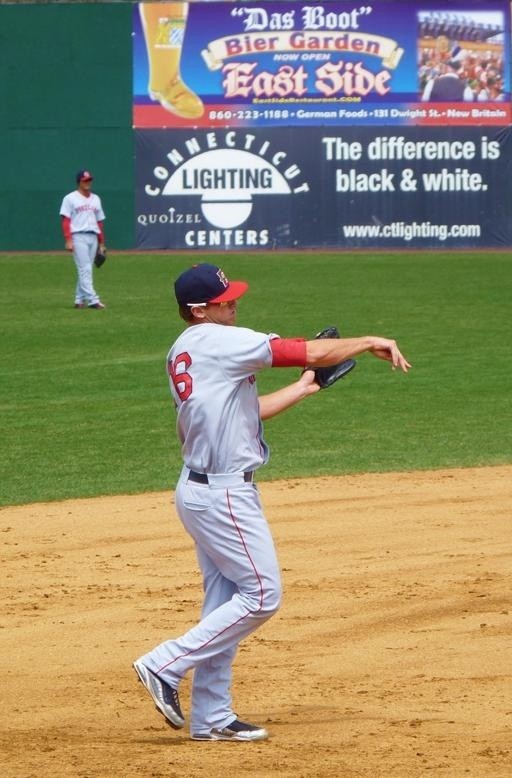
[{"xmin": 216, "ymin": 301, "xmax": 228, "ymax": 309}]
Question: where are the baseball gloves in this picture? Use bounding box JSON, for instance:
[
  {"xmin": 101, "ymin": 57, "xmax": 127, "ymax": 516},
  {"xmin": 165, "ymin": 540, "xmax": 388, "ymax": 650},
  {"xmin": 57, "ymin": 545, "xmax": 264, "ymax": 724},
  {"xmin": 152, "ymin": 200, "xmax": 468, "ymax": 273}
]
[
  {"xmin": 94, "ymin": 248, "xmax": 107, "ymax": 268},
  {"xmin": 301, "ymin": 327, "xmax": 356, "ymax": 390}
]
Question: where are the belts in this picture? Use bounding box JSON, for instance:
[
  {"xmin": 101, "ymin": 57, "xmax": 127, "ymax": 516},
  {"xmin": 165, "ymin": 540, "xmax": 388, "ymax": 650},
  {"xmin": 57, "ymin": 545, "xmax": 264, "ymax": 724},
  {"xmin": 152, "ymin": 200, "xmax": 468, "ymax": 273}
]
[{"xmin": 184, "ymin": 471, "xmax": 253, "ymax": 484}]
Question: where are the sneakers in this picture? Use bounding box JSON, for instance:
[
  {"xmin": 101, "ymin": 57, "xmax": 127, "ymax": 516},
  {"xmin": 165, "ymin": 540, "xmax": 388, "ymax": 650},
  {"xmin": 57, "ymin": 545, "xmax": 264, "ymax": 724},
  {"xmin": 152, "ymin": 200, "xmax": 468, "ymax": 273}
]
[
  {"xmin": 87, "ymin": 302, "xmax": 104, "ymax": 310},
  {"xmin": 190, "ymin": 720, "xmax": 269, "ymax": 742},
  {"xmin": 75, "ymin": 303, "xmax": 83, "ymax": 309},
  {"xmin": 131, "ymin": 658, "xmax": 185, "ymax": 730}
]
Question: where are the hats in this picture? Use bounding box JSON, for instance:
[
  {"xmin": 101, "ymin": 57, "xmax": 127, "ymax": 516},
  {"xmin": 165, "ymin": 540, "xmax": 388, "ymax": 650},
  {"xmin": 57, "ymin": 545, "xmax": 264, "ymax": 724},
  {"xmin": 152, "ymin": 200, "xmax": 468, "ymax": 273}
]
[
  {"xmin": 76, "ymin": 169, "xmax": 93, "ymax": 184},
  {"xmin": 174, "ymin": 262, "xmax": 248, "ymax": 308}
]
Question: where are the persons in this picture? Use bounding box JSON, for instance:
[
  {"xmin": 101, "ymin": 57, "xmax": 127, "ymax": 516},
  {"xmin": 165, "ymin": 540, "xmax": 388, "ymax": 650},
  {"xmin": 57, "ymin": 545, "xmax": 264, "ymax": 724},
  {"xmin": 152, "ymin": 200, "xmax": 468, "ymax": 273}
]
[
  {"xmin": 56, "ymin": 168, "xmax": 112, "ymax": 311},
  {"xmin": 129, "ymin": 260, "xmax": 413, "ymax": 742},
  {"xmin": 418, "ymin": 45, "xmax": 505, "ymax": 103}
]
